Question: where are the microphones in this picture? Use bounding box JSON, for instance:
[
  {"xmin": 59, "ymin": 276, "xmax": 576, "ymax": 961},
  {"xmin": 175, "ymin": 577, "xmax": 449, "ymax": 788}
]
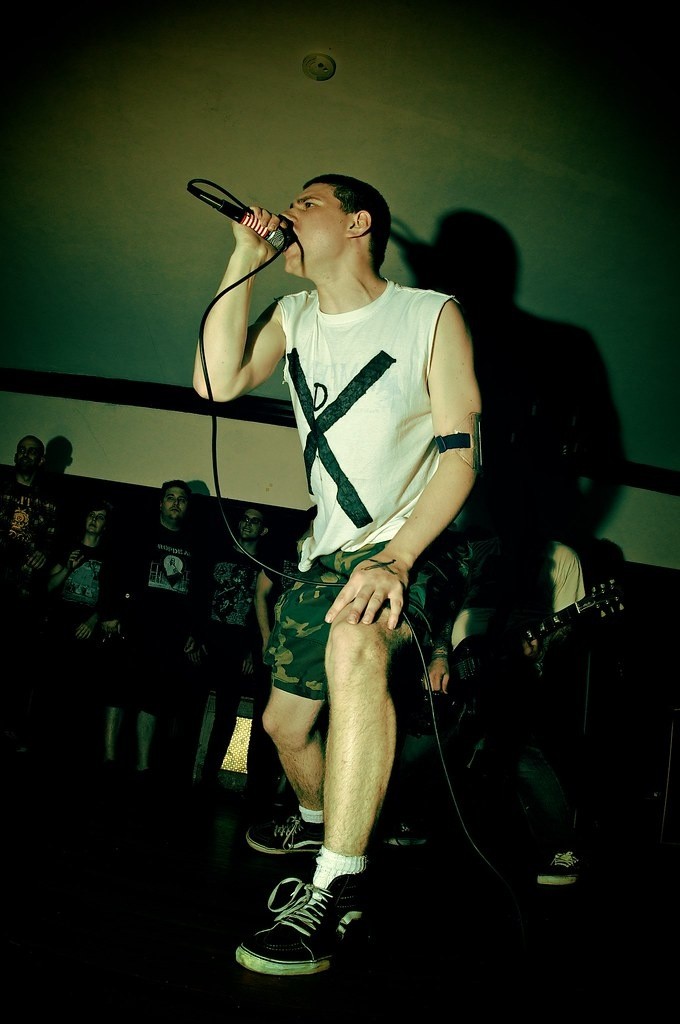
[{"xmin": 186, "ymin": 185, "xmax": 292, "ymax": 253}]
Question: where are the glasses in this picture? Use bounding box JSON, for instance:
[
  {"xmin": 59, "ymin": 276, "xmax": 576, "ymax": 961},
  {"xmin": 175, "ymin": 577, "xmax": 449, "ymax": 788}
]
[{"xmin": 241, "ymin": 514, "xmax": 264, "ymax": 524}]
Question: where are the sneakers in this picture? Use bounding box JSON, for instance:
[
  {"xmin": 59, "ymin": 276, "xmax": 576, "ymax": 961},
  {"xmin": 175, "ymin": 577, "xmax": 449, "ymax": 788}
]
[
  {"xmin": 246, "ymin": 809, "xmax": 326, "ymax": 854},
  {"xmin": 536, "ymin": 851, "xmax": 581, "ymax": 887},
  {"xmin": 235, "ymin": 870, "xmax": 364, "ymax": 977}
]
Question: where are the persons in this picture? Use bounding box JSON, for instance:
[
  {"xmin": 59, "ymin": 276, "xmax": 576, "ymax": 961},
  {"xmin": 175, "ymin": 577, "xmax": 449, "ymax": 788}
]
[
  {"xmin": 191, "ymin": 174, "xmax": 486, "ymax": 977},
  {"xmin": 0, "ymin": 432, "xmax": 590, "ymax": 887}
]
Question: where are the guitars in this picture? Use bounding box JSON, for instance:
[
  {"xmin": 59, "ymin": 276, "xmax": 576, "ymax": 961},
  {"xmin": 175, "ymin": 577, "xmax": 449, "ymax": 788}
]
[{"xmin": 393, "ymin": 578, "xmax": 627, "ymax": 773}]
